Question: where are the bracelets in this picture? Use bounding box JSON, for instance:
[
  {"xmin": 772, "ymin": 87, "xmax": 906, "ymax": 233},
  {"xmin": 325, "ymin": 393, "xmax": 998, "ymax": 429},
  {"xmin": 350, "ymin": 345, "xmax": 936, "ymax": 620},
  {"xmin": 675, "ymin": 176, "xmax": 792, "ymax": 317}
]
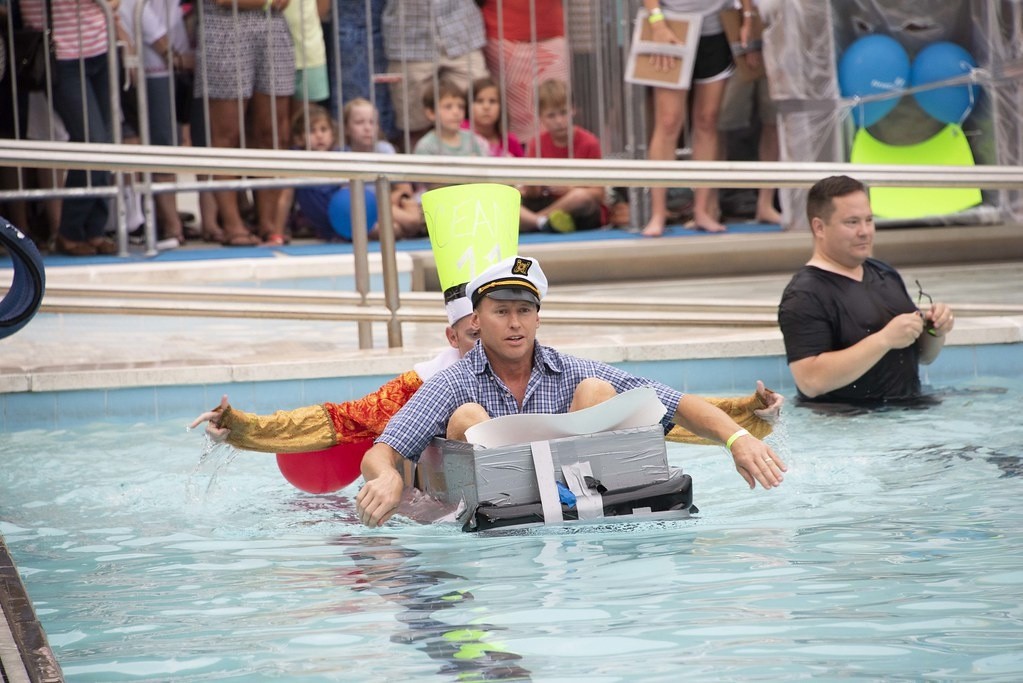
[
  {"xmin": 744, "ymin": 11, "xmax": 755, "ymax": 17},
  {"xmin": 647, "ymin": 14, "xmax": 664, "ymax": 23},
  {"xmin": 727, "ymin": 428, "xmax": 749, "ymax": 449},
  {"xmin": 162, "ymin": 45, "xmax": 184, "ymax": 72}
]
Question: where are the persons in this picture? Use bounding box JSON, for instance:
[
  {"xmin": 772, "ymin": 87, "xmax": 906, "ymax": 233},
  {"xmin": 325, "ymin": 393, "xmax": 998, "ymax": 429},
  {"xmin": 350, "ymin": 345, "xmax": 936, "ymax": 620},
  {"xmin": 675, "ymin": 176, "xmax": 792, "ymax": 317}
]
[
  {"xmin": 358, "ymin": 257, "xmax": 788, "ymax": 526},
  {"xmin": 776, "ymin": 174, "xmax": 954, "ymax": 400},
  {"xmin": 4, "ymin": 0, "xmax": 787, "ymax": 254},
  {"xmin": 188, "ymin": 182, "xmax": 781, "ymax": 451}
]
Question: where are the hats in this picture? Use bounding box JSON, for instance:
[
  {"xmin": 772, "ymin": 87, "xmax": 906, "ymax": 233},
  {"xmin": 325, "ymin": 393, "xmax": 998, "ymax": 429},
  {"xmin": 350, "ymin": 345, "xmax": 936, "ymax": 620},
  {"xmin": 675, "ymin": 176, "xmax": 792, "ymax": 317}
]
[
  {"xmin": 465, "ymin": 256, "xmax": 549, "ymax": 306},
  {"xmin": 420, "ymin": 183, "xmax": 521, "ymax": 328}
]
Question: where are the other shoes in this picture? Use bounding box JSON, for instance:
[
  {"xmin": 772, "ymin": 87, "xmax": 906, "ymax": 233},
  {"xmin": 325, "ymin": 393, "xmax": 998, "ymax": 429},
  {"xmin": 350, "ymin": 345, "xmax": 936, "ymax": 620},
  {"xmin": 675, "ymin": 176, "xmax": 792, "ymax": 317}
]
[
  {"xmin": 549, "ymin": 210, "xmax": 576, "ymax": 233},
  {"xmin": 85, "ymin": 237, "xmax": 118, "ymax": 254},
  {"xmin": 57, "ymin": 238, "xmax": 96, "ymax": 255}
]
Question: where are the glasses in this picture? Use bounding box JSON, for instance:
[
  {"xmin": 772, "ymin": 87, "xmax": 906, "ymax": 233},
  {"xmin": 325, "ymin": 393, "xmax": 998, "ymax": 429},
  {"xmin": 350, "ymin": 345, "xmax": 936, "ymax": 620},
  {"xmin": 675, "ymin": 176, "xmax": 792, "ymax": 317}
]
[{"xmin": 915, "ymin": 280, "xmax": 940, "ymax": 338}]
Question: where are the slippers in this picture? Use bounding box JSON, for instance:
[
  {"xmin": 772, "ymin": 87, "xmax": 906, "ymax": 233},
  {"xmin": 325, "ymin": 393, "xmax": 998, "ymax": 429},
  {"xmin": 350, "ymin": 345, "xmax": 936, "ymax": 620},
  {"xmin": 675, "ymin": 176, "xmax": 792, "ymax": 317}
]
[
  {"xmin": 164, "ymin": 231, "xmax": 185, "ymax": 246},
  {"xmin": 203, "ymin": 229, "xmax": 220, "ymax": 241},
  {"xmin": 262, "ymin": 231, "xmax": 292, "ymax": 244},
  {"xmin": 220, "ymin": 232, "xmax": 261, "ymax": 246}
]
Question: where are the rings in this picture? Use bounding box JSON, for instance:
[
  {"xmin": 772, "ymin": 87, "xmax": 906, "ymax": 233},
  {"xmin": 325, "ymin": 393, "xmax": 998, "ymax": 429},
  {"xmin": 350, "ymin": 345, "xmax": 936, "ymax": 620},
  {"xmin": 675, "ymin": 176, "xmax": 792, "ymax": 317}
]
[{"xmin": 765, "ymin": 458, "xmax": 772, "ymax": 462}]
